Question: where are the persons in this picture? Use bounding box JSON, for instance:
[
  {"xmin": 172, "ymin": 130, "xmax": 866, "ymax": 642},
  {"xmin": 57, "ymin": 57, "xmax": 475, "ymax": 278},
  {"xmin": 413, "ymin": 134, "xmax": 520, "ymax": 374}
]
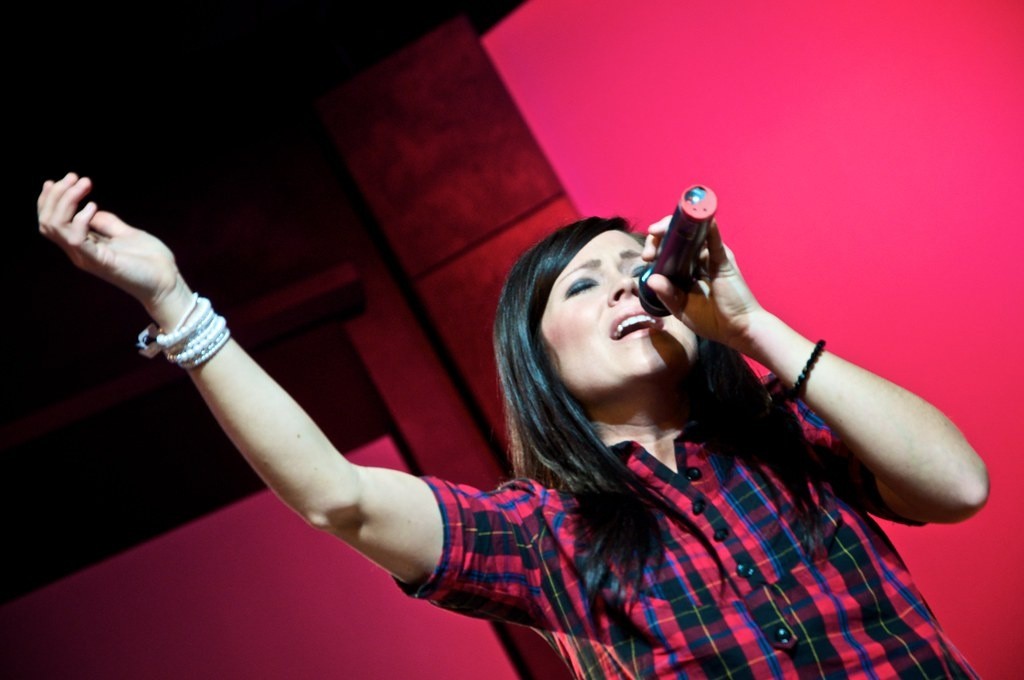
[{"xmin": 36, "ymin": 172, "xmax": 988, "ymax": 680}]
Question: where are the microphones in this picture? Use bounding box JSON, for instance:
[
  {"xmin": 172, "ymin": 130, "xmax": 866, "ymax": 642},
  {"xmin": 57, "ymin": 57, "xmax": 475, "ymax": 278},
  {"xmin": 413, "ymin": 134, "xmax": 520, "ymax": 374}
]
[{"xmin": 638, "ymin": 185, "xmax": 718, "ymax": 318}]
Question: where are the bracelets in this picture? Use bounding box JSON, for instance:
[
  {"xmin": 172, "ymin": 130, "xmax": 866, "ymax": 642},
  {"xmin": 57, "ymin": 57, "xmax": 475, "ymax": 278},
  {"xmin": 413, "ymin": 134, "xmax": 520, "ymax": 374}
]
[
  {"xmin": 792, "ymin": 339, "xmax": 826, "ymax": 396},
  {"xmin": 137, "ymin": 292, "xmax": 230, "ymax": 370}
]
